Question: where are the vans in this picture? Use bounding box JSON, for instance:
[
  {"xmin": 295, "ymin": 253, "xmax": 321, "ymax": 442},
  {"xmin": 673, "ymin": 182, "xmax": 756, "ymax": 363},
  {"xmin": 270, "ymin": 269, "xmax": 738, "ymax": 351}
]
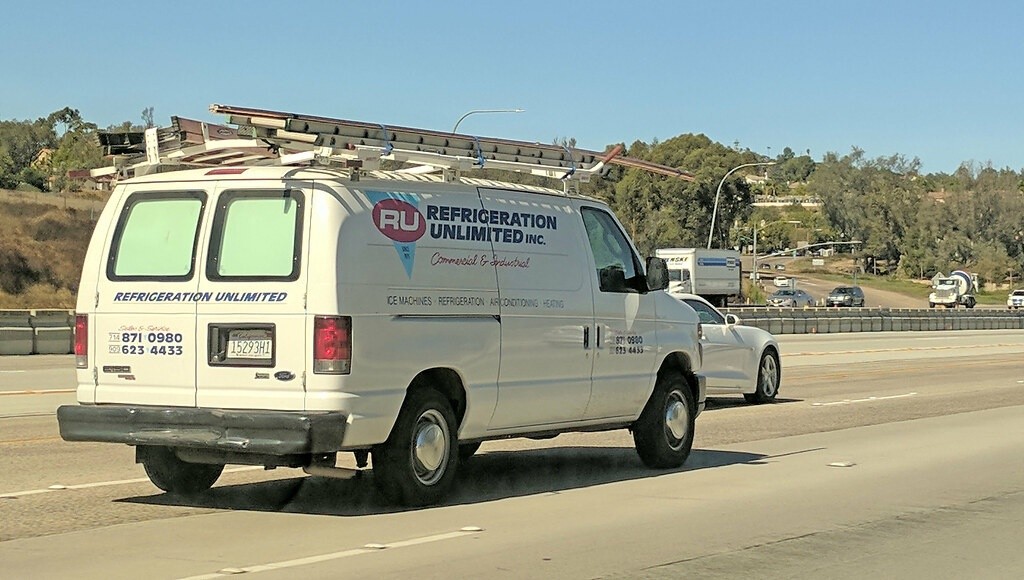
[{"xmin": 57, "ymin": 143, "xmax": 707, "ymax": 509}]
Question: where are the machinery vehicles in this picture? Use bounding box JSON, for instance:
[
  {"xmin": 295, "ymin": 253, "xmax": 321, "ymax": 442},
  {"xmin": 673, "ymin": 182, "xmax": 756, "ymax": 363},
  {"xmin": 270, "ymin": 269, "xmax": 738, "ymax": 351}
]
[{"xmin": 928, "ymin": 270, "xmax": 977, "ymax": 309}]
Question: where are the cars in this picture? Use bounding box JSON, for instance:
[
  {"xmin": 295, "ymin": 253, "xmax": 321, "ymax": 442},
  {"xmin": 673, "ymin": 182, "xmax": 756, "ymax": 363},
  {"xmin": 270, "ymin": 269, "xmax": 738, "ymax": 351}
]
[
  {"xmin": 765, "ymin": 289, "xmax": 816, "ymax": 307},
  {"xmin": 759, "ymin": 263, "xmax": 770, "ymax": 269},
  {"xmin": 1007, "ymin": 290, "xmax": 1024, "ymax": 309},
  {"xmin": 775, "ymin": 263, "xmax": 785, "ymax": 271},
  {"xmin": 670, "ymin": 294, "xmax": 782, "ymax": 405},
  {"xmin": 774, "ymin": 277, "xmax": 789, "ymax": 286}
]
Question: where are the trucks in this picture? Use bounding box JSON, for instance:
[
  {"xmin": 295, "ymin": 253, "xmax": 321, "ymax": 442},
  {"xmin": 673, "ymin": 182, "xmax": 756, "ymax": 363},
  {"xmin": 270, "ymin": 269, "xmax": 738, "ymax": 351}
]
[{"xmin": 655, "ymin": 249, "xmax": 740, "ymax": 306}]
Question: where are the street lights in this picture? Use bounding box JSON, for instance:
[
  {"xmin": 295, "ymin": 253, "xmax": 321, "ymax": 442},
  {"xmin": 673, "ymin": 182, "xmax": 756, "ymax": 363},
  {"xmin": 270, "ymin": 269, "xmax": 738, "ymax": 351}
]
[
  {"xmin": 707, "ymin": 162, "xmax": 777, "ymax": 249},
  {"xmin": 754, "ymin": 220, "xmax": 801, "ymax": 287},
  {"xmin": 452, "ymin": 109, "xmax": 527, "ymax": 135}
]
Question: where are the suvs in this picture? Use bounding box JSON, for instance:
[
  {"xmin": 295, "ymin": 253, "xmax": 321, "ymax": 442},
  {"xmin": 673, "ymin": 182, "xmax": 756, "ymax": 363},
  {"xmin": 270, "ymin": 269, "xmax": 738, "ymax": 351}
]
[{"xmin": 828, "ymin": 285, "xmax": 865, "ymax": 307}]
